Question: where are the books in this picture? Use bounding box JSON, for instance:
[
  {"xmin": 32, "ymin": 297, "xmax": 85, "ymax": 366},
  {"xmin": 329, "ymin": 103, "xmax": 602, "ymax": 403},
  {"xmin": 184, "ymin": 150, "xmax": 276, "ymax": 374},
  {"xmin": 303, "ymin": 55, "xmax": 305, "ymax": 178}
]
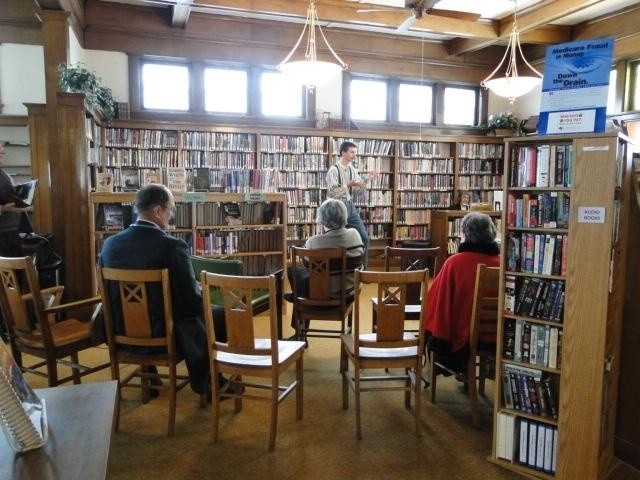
[
  {"xmin": 448, "ymin": 142, "xmax": 504, "ymax": 255},
  {"xmin": 333, "ymin": 136, "xmax": 395, "ymax": 238},
  {"xmin": 85, "ymin": 119, "xmax": 257, "ymax": 194},
  {"xmin": 399, "ymin": 139, "xmax": 455, "ymax": 242},
  {"xmin": 260, "ymin": 134, "xmax": 329, "ymax": 262},
  {"xmin": 495, "ymin": 144, "xmax": 574, "ymax": 476},
  {"xmin": 0, "ymin": 178, "xmax": 40, "ymax": 207},
  {"xmin": 97, "ymin": 202, "xmax": 283, "ymax": 292}
]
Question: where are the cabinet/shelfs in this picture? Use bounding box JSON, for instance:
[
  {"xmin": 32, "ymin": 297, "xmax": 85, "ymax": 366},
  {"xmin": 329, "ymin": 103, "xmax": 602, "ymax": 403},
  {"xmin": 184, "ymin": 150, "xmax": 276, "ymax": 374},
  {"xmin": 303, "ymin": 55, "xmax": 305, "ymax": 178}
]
[
  {"xmin": 0, "ymin": 103, "xmax": 50, "ymax": 235},
  {"xmin": 97, "ymin": 117, "xmax": 508, "ymax": 267},
  {"xmin": 88, "ymin": 192, "xmax": 287, "ymax": 317},
  {"xmin": 55, "ymin": 94, "xmax": 104, "ymax": 320},
  {"xmin": 431, "ymin": 209, "xmax": 502, "ymax": 279},
  {"xmin": 486, "ymin": 132, "xmax": 633, "ymax": 479}
]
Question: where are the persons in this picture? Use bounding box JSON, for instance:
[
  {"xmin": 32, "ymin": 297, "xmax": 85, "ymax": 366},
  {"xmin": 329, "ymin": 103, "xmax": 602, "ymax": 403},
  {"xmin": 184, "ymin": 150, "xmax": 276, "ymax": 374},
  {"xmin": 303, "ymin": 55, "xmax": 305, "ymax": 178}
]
[
  {"xmin": 284, "ymin": 198, "xmax": 363, "ymax": 348},
  {"xmin": 95, "ymin": 184, "xmax": 245, "ymax": 402},
  {"xmin": 423, "ymin": 212, "xmax": 501, "ymax": 387},
  {"xmin": 325, "ymin": 141, "xmax": 379, "ymax": 269},
  {"xmin": 0, "ymin": 146, "xmax": 24, "ymax": 345}
]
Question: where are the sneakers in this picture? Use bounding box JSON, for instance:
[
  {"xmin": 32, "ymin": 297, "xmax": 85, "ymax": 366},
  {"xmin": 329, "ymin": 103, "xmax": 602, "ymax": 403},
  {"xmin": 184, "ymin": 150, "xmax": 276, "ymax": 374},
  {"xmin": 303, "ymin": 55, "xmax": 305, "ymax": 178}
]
[
  {"xmin": 141, "ymin": 364, "xmax": 161, "ymax": 400},
  {"xmin": 283, "ymin": 292, "xmax": 293, "ymax": 303},
  {"xmin": 199, "ymin": 373, "xmax": 247, "ymax": 405},
  {"xmin": 282, "ymin": 334, "xmax": 309, "ymax": 349}
]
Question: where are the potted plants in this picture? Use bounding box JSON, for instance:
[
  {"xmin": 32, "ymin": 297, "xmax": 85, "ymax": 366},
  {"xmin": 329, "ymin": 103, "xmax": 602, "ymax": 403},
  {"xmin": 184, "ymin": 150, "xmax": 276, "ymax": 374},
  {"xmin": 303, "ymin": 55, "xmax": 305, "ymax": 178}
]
[
  {"xmin": 57, "ymin": 64, "xmax": 98, "ymax": 109},
  {"xmin": 478, "ymin": 122, "xmax": 495, "ymax": 137},
  {"xmin": 488, "ymin": 111, "xmax": 523, "ymax": 138}
]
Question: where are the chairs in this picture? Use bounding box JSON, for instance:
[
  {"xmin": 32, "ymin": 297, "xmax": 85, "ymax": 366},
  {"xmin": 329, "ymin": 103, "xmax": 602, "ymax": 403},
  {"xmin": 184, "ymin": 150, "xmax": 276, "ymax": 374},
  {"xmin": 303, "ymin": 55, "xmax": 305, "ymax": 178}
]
[
  {"xmin": 339, "ymin": 268, "xmax": 431, "ymax": 441},
  {"xmin": 199, "ymin": 272, "xmax": 307, "ymax": 453},
  {"xmin": 190, "ymin": 255, "xmax": 270, "ymax": 317},
  {"xmin": 0, "ymin": 254, "xmax": 111, "ymax": 388},
  {"xmin": 289, "ymin": 246, "xmax": 353, "ymax": 372},
  {"xmin": 427, "ymin": 264, "xmax": 500, "ymax": 430},
  {"xmin": 95, "ymin": 267, "xmax": 207, "ymax": 437},
  {"xmin": 370, "ymin": 245, "xmax": 440, "ymax": 372},
  {"xmin": 5, "ymin": 286, "xmax": 65, "ymax": 330}
]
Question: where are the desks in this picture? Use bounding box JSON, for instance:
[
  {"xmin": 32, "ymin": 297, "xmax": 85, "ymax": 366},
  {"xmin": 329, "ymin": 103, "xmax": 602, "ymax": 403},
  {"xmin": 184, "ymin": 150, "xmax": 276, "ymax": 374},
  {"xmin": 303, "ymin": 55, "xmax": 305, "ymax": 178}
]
[{"xmin": 0, "ymin": 380, "xmax": 119, "ymax": 480}]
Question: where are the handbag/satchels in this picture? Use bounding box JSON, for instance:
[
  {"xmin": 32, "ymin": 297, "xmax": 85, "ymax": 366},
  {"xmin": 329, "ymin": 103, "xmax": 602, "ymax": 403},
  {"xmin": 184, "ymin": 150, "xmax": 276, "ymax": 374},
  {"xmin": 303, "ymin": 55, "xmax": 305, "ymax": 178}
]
[{"xmin": 12, "ymin": 230, "xmax": 65, "ymax": 281}]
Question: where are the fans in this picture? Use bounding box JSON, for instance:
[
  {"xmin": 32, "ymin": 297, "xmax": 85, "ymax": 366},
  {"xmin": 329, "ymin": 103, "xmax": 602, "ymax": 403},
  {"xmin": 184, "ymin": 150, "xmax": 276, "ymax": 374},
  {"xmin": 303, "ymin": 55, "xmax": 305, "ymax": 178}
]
[{"xmin": 355, "ymin": 0, "xmax": 481, "ymax": 37}]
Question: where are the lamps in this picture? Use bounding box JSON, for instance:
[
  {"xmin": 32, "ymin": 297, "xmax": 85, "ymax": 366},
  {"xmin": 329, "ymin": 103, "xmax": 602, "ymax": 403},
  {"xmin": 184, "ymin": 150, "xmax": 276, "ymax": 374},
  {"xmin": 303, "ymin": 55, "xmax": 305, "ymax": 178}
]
[
  {"xmin": 277, "ymin": 0, "xmax": 349, "ymax": 94},
  {"xmin": 479, "ymin": 0, "xmax": 543, "ymax": 120}
]
